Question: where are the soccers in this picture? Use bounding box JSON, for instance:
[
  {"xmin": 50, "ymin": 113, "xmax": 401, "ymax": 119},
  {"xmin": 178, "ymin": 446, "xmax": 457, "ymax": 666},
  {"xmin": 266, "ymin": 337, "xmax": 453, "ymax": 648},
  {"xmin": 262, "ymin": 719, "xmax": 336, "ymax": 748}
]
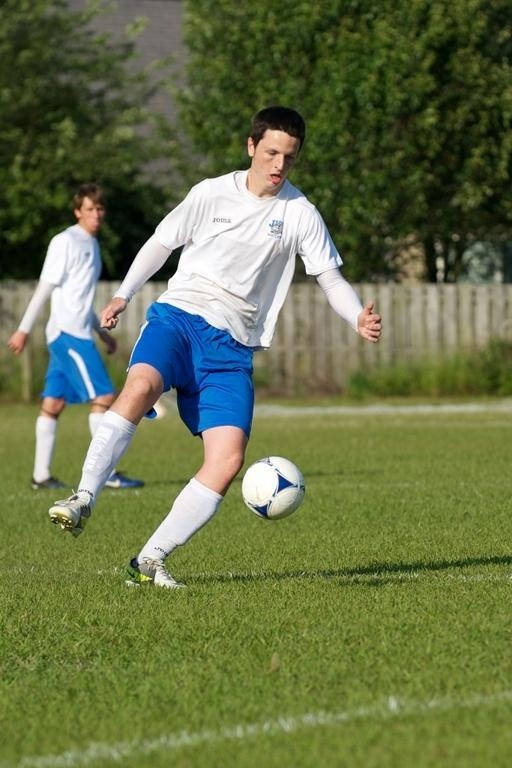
[{"xmin": 240, "ymin": 457, "xmax": 307, "ymax": 521}]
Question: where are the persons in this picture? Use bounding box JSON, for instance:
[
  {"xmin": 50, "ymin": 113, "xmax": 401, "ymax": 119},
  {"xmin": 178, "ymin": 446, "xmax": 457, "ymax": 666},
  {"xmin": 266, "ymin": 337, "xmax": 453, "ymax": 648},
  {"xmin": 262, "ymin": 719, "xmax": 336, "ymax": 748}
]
[
  {"xmin": 5, "ymin": 183, "xmax": 145, "ymax": 492},
  {"xmin": 47, "ymin": 106, "xmax": 383, "ymax": 590}
]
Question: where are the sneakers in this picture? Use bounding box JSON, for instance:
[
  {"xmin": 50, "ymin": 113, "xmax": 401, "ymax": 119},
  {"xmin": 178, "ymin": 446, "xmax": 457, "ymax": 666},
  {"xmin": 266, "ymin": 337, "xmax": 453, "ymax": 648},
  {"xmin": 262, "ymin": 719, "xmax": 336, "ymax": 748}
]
[
  {"xmin": 105, "ymin": 470, "xmax": 145, "ymax": 488},
  {"xmin": 123, "ymin": 558, "xmax": 187, "ymax": 589},
  {"xmin": 48, "ymin": 492, "xmax": 94, "ymax": 538},
  {"xmin": 30, "ymin": 476, "xmax": 72, "ymax": 490}
]
